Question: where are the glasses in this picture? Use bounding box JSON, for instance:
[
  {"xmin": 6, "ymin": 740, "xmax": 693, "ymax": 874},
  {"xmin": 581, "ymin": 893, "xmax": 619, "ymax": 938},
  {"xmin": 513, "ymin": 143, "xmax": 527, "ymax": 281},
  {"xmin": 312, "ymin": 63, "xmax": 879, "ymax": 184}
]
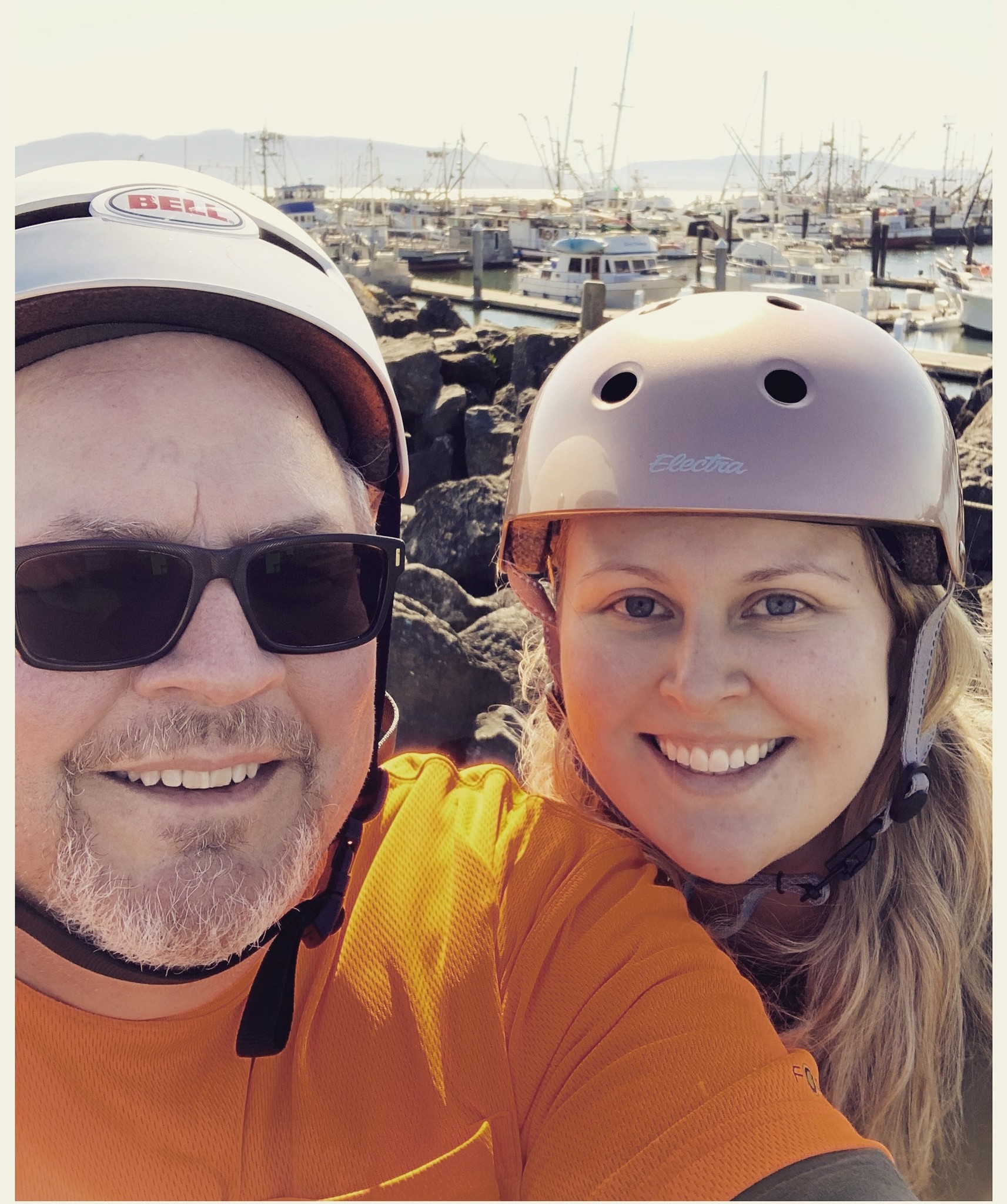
[{"xmin": 14, "ymin": 532, "xmax": 405, "ymax": 671}]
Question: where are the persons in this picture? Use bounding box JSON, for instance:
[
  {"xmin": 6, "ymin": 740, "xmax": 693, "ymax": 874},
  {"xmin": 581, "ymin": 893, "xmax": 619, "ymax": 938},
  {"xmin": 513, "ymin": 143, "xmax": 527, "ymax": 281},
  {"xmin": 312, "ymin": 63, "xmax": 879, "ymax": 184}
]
[
  {"xmin": 474, "ymin": 292, "xmax": 993, "ymax": 1202},
  {"xmin": 15, "ymin": 162, "xmax": 912, "ymax": 1201}
]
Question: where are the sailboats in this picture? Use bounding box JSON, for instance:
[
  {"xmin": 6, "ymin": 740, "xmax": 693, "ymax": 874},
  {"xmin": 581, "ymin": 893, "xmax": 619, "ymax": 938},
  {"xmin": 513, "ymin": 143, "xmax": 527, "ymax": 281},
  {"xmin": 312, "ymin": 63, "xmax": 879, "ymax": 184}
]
[{"xmin": 246, "ymin": 22, "xmax": 994, "ymax": 268}]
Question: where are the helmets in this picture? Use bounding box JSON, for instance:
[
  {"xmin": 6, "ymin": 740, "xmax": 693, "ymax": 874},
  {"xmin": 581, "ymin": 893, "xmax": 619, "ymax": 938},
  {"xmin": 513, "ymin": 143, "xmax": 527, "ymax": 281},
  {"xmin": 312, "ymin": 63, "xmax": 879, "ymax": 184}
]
[
  {"xmin": 496, "ymin": 288, "xmax": 968, "ymax": 590},
  {"xmin": 17, "ymin": 158, "xmax": 414, "ymax": 497}
]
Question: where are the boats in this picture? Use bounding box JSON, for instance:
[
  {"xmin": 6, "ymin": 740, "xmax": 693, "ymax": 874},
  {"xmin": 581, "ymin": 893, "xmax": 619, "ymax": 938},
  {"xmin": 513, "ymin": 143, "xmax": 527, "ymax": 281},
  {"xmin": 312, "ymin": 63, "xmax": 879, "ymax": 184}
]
[
  {"xmin": 698, "ymin": 234, "xmax": 892, "ymax": 313},
  {"xmin": 933, "ymin": 262, "xmax": 993, "ymax": 334},
  {"xmin": 517, "ymin": 231, "xmax": 691, "ymax": 309}
]
